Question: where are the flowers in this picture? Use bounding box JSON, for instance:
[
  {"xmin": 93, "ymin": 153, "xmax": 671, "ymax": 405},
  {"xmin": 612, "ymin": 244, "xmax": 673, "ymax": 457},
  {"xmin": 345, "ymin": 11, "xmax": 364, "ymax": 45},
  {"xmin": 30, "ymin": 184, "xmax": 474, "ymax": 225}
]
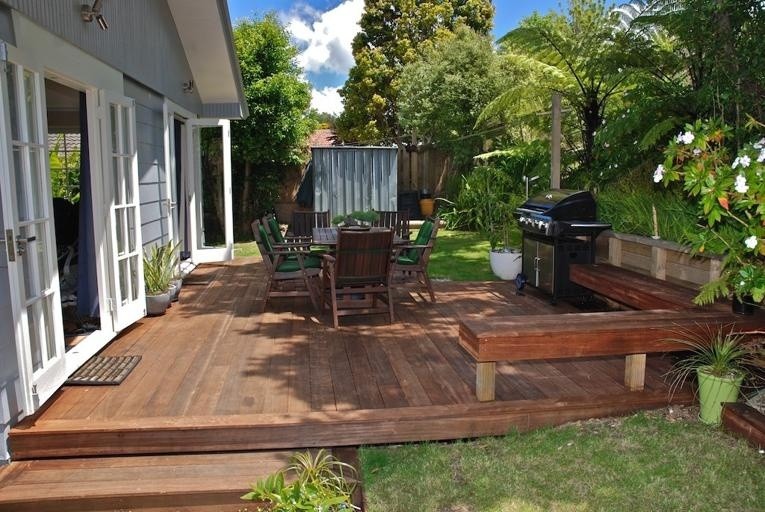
[{"xmin": 653, "ymin": 112, "xmax": 765, "ymax": 305}]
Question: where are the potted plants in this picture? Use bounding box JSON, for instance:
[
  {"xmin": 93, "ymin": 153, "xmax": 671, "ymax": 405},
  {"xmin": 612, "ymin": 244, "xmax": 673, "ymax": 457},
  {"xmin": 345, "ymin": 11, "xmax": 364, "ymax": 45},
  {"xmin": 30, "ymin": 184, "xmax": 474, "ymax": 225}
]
[
  {"xmin": 433, "ymin": 166, "xmax": 528, "ymax": 280},
  {"xmin": 648, "ymin": 315, "xmax": 765, "ymax": 426},
  {"xmin": 143, "ymin": 237, "xmax": 200, "ymax": 315}
]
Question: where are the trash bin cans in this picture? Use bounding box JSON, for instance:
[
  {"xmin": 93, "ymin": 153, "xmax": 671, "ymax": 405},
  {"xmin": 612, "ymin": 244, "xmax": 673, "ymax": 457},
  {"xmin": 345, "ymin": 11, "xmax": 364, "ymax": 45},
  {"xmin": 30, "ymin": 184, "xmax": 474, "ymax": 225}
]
[{"xmin": 513, "ymin": 188, "xmax": 612, "ymax": 305}]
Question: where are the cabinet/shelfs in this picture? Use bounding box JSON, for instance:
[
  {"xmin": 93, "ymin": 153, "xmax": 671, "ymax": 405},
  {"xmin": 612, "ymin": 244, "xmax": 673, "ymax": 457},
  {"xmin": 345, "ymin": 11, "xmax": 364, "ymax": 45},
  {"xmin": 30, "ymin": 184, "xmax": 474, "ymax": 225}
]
[{"xmin": 523, "ymin": 238, "xmax": 591, "ymax": 297}]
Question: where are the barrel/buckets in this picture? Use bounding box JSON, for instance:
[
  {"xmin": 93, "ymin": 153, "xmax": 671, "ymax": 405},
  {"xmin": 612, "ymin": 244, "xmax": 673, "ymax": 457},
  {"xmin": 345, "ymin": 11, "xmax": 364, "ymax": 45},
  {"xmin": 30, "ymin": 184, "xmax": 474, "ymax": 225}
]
[{"xmin": 697, "ymin": 363, "xmax": 745, "ymax": 423}]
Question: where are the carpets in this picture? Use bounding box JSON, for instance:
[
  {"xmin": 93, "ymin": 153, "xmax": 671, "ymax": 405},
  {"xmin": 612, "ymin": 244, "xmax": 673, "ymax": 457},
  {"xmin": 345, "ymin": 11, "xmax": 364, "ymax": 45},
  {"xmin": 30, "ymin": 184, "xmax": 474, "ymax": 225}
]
[
  {"xmin": 183, "ymin": 274, "xmax": 216, "ymax": 285},
  {"xmin": 66, "ymin": 355, "xmax": 143, "ymax": 385}
]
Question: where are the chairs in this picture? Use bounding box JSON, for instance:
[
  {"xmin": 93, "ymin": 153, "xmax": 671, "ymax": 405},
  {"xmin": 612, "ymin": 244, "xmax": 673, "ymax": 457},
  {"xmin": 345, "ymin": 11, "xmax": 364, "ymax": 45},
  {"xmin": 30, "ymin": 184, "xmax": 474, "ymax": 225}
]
[{"xmin": 251, "ymin": 208, "xmax": 441, "ymax": 329}]
